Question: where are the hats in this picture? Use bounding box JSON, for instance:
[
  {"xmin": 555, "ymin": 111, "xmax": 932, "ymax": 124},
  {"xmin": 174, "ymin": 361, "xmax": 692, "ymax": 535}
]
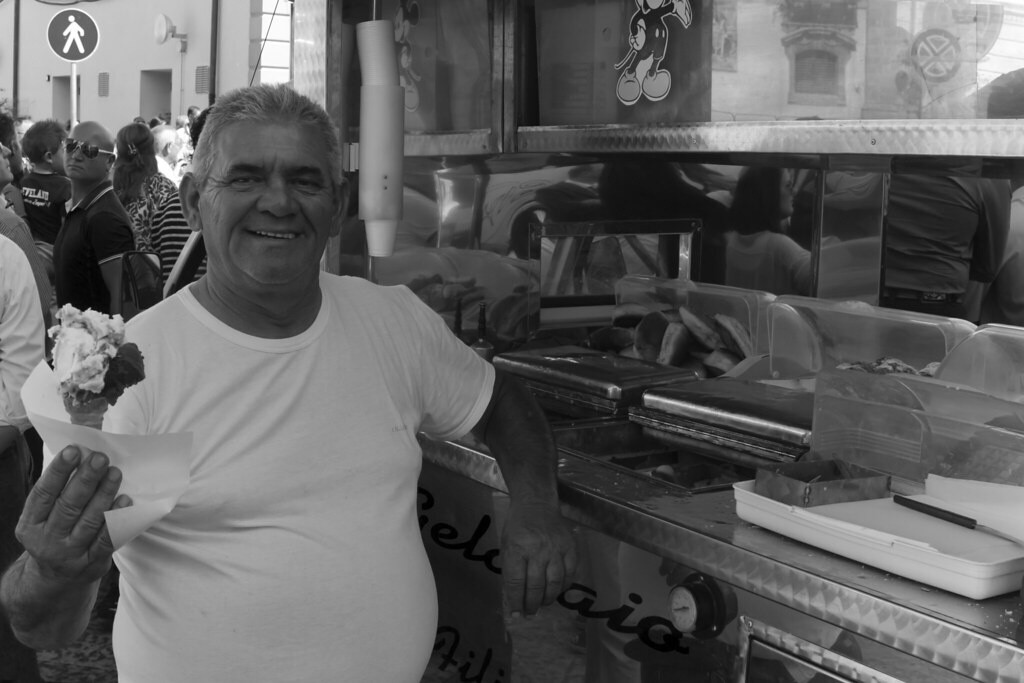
[
  {"xmin": 149, "ymin": 117, "xmax": 167, "ymax": 130},
  {"xmin": 134, "ymin": 117, "xmax": 146, "ymax": 124}
]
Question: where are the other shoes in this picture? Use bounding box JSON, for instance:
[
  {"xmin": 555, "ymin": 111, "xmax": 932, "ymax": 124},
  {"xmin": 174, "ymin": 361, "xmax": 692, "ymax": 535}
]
[{"xmin": 87, "ymin": 584, "xmax": 120, "ymax": 631}]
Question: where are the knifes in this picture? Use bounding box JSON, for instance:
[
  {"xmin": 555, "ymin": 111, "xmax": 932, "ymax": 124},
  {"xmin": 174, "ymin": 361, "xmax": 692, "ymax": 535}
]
[{"xmin": 893, "ymin": 494, "xmax": 1024, "ymax": 548}]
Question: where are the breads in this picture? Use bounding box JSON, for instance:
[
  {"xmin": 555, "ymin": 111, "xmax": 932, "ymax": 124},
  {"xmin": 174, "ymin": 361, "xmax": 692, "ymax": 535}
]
[
  {"xmin": 582, "ymin": 301, "xmax": 756, "ymax": 380},
  {"xmin": 485, "ymin": 293, "xmax": 539, "ymax": 343}
]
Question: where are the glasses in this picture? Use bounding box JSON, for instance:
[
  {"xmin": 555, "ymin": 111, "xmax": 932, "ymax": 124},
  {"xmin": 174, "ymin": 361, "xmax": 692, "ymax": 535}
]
[{"xmin": 64, "ymin": 137, "xmax": 115, "ymax": 160}]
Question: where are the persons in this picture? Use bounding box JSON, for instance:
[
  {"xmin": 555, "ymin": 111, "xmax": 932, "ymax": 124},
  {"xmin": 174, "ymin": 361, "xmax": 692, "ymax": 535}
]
[
  {"xmin": 0, "ymin": 113, "xmax": 53, "ymax": 683},
  {"xmin": 51, "ymin": 121, "xmax": 132, "ymax": 318},
  {"xmin": 134, "ymin": 106, "xmax": 200, "ymax": 181},
  {"xmin": 19, "ymin": 120, "xmax": 70, "ymax": 244},
  {"xmin": 151, "ymin": 107, "xmax": 210, "ymax": 291},
  {"xmin": 113, "ymin": 123, "xmax": 176, "ymax": 250},
  {"xmin": 598, "ymin": 160, "xmax": 812, "ymax": 296},
  {"xmin": 882, "ymin": 173, "xmax": 1024, "ymax": 318},
  {"xmin": 0, "ymin": 85, "xmax": 578, "ymax": 683}
]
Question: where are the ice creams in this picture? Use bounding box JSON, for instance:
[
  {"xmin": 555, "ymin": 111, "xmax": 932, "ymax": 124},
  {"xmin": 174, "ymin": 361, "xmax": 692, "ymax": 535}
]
[{"xmin": 47, "ymin": 302, "xmax": 146, "ymax": 431}]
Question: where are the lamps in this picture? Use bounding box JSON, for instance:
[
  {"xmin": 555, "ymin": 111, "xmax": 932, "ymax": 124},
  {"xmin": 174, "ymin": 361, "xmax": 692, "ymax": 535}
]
[{"xmin": 153, "ymin": 13, "xmax": 187, "ymax": 44}]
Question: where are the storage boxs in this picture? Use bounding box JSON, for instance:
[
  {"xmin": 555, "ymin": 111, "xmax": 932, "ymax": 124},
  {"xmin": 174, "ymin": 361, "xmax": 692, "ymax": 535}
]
[{"xmin": 761, "ymin": 457, "xmax": 891, "ymax": 509}]
